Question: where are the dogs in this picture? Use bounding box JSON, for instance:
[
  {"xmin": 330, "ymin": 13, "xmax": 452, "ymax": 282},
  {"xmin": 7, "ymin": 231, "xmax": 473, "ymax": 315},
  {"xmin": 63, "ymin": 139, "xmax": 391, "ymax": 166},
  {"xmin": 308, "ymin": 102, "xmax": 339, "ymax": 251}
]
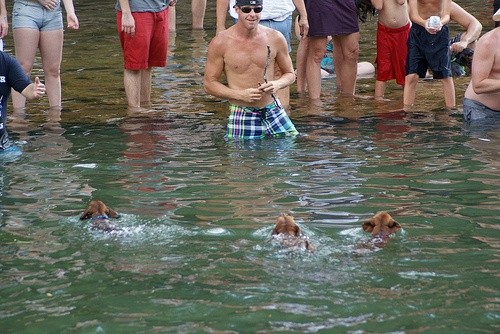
[
  {"xmin": 362, "ymin": 211, "xmax": 401, "ymax": 249},
  {"xmin": 271, "ymin": 216, "xmax": 313, "ymax": 251},
  {"xmin": 79, "ymin": 201, "xmax": 119, "ymax": 231}
]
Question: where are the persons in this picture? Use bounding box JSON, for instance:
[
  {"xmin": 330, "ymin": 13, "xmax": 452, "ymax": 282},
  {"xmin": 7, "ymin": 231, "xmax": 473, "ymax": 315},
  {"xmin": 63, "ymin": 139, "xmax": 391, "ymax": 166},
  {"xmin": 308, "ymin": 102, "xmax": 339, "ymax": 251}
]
[
  {"xmin": 11, "ymin": 0, "xmax": 79, "ymax": 123},
  {"xmin": 168, "ymin": 0, "xmax": 500, "ymax": 147},
  {"xmin": 0, "ymin": 49, "xmax": 45, "ymax": 165},
  {"xmin": 116, "ymin": 0, "xmax": 170, "ymax": 119},
  {"xmin": 0, "ymin": 0, "xmax": 8, "ymax": 51}
]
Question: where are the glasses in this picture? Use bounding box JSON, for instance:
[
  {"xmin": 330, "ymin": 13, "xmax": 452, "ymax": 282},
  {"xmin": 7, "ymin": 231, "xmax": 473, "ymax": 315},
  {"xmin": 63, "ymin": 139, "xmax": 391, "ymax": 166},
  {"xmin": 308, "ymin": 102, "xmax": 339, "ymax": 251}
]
[{"xmin": 239, "ymin": 5, "xmax": 263, "ymax": 14}]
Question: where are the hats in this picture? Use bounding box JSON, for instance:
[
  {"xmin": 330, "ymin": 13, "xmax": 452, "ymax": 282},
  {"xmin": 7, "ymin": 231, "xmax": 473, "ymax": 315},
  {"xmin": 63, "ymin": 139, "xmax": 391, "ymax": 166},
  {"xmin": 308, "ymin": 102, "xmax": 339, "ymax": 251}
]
[{"xmin": 233, "ymin": 0, "xmax": 264, "ymax": 9}]
